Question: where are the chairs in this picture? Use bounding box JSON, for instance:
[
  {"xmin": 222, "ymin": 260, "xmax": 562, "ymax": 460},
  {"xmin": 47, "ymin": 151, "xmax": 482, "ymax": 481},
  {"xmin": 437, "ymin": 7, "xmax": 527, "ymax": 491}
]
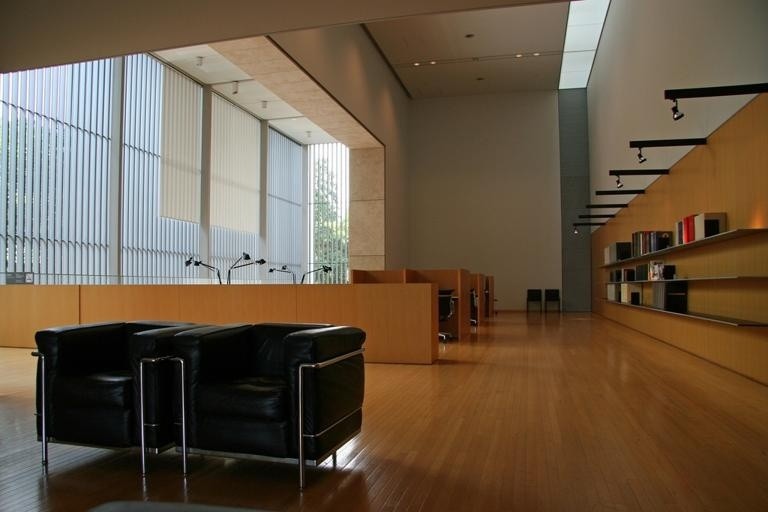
[
  {"xmin": 438, "ymin": 289, "xmax": 456, "ymax": 342},
  {"xmin": 470, "ymin": 288, "xmax": 477, "ymax": 325},
  {"xmin": 527, "ymin": 289, "xmax": 542, "ymax": 312},
  {"xmin": 170, "ymin": 322, "xmax": 367, "ymax": 491},
  {"xmin": 31, "ymin": 319, "xmax": 216, "ymax": 477},
  {"xmin": 545, "ymin": 290, "xmax": 560, "ymax": 312}
]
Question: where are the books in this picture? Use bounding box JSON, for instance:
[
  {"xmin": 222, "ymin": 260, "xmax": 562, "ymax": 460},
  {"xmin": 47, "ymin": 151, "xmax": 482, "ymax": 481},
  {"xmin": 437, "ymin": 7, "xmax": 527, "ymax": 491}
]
[{"xmin": 602, "ymin": 209, "xmax": 728, "ymax": 314}]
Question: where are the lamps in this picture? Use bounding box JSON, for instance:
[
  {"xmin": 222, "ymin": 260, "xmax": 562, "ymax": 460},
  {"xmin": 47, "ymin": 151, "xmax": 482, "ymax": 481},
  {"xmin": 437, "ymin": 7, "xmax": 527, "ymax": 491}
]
[
  {"xmin": 227, "ymin": 252, "xmax": 266, "ymax": 285},
  {"xmin": 616, "ymin": 175, "xmax": 623, "ymax": 188},
  {"xmin": 671, "ymin": 99, "xmax": 684, "ymax": 121},
  {"xmin": 638, "ymin": 148, "xmax": 647, "ymax": 164},
  {"xmin": 185, "ymin": 257, "xmax": 222, "ymax": 285},
  {"xmin": 268, "ymin": 265, "xmax": 297, "ymax": 284},
  {"xmin": 301, "ymin": 266, "xmax": 332, "ymax": 284},
  {"xmin": 573, "ymin": 226, "xmax": 579, "ymax": 234}
]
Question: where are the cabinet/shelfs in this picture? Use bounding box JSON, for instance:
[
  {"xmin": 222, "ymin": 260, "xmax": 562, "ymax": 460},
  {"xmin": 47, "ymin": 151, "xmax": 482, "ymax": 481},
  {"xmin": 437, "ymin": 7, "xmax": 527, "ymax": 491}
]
[{"xmin": 599, "ymin": 227, "xmax": 768, "ymax": 327}]
